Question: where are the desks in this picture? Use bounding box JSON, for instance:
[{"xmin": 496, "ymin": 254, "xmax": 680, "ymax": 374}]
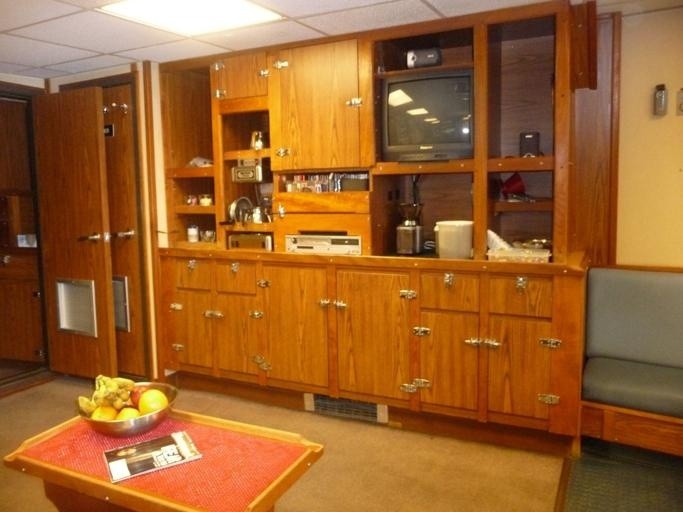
[{"xmin": 1, "ymin": 407, "xmax": 326, "ymax": 512}]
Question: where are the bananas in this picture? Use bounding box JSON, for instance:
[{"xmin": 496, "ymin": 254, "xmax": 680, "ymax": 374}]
[{"xmin": 93, "ymin": 375, "xmax": 135, "ymax": 401}]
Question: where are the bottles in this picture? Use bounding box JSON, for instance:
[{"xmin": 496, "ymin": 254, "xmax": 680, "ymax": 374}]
[{"xmin": 187, "ymin": 224, "xmax": 199, "ymax": 243}]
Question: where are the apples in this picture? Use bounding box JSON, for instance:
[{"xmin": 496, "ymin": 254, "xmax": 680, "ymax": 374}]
[{"xmin": 130, "ymin": 387, "xmax": 147, "ymax": 407}]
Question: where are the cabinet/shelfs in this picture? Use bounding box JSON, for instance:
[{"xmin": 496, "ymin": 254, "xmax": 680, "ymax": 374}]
[
  {"xmin": 174, "ymin": 258, "xmax": 258, "ymax": 369},
  {"xmin": 215, "ymin": 51, "xmax": 269, "ymax": 103},
  {"xmin": 416, "ymin": 276, "xmax": 552, "ymax": 420},
  {"xmin": 336, "ymin": 270, "xmax": 410, "ymax": 404},
  {"xmin": 166, "ymin": 62, "xmax": 221, "ymax": 246},
  {"xmin": 373, "ymin": 26, "xmax": 474, "ymax": 256},
  {"xmin": 274, "ymin": 175, "xmax": 374, "ymax": 257},
  {"xmin": 274, "ymin": 39, "xmax": 361, "ymax": 172},
  {"xmin": 484, "ymin": 16, "xmax": 556, "ymax": 261},
  {"xmin": 258, "ymin": 265, "xmax": 329, "ymax": 388},
  {"xmin": 223, "ymin": 110, "xmax": 272, "ymax": 251}
]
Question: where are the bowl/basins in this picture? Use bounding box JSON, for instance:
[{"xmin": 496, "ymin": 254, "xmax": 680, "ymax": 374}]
[{"xmin": 79, "ymin": 380, "xmax": 180, "ymax": 438}]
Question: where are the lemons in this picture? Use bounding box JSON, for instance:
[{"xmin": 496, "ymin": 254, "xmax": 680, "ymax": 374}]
[
  {"xmin": 139, "ymin": 389, "xmax": 168, "ymax": 414},
  {"xmin": 115, "ymin": 407, "xmax": 139, "ymax": 420},
  {"xmin": 90, "ymin": 405, "xmax": 117, "ymax": 422}
]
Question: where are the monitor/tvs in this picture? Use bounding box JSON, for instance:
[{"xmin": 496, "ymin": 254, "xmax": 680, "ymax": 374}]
[{"xmin": 380, "ymin": 68, "xmax": 474, "ymax": 162}]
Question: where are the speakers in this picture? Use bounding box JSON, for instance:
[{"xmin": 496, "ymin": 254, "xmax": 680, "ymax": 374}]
[{"xmin": 519, "ymin": 131, "xmax": 539, "ymax": 158}]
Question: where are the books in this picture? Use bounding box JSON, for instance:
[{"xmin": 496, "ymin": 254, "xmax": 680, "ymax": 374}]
[{"xmin": 102, "ymin": 429, "xmax": 202, "ymax": 484}]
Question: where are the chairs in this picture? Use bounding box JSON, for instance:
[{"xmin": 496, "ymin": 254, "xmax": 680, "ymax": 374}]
[{"xmin": 579, "ymin": 260, "xmax": 683, "ymax": 459}]
